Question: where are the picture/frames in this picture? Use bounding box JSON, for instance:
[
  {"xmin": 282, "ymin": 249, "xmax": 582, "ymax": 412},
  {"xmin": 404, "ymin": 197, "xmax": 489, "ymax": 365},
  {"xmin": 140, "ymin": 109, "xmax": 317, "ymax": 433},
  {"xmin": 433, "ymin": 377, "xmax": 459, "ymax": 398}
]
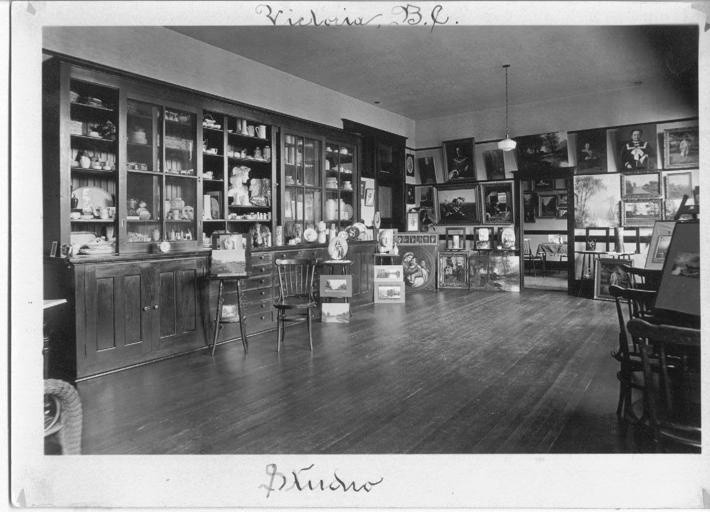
[
  {"xmin": 319, "ymin": 274, "xmax": 352, "ymax": 298},
  {"xmin": 320, "ymin": 302, "xmax": 350, "ymax": 323},
  {"xmin": 361, "ymin": 181, "xmax": 366, "ymax": 199},
  {"xmin": 365, "ymin": 188, "xmax": 374, "ymax": 206},
  {"xmin": 373, "ymin": 265, "xmax": 403, "ymax": 283},
  {"xmin": 374, "ymin": 282, "xmax": 406, "ymax": 304}
]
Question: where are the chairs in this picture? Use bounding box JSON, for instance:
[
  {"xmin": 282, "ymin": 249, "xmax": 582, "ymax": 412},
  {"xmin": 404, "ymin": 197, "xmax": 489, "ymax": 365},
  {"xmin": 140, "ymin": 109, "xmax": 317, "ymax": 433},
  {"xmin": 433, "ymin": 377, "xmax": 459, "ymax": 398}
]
[
  {"xmin": 273, "ymin": 256, "xmax": 317, "ymax": 352},
  {"xmin": 43, "ymin": 379, "xmax": 83, "ymax": 455},
  {"xmin": 608, "ymin": 261, "xmax": 702, "ymax": 454}
]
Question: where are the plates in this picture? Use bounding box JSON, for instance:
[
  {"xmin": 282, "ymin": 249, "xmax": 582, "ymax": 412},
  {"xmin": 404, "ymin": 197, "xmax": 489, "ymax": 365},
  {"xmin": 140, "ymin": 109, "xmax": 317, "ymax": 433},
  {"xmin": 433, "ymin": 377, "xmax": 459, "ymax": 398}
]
[
  {"xmin": 283, "ymin": 134, "xmax": 369, "ymax": 245},
  {"xmin": 327, "ymin": 237, "xmax": 349, "ymax": 259}
]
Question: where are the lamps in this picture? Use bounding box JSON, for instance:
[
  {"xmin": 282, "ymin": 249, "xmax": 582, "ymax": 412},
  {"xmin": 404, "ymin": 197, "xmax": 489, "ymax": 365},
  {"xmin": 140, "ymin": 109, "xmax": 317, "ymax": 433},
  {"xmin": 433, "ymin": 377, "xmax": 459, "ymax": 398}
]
[{"xmin": 496, "ymin": 64, "xmax": 516, "ymax": 152}]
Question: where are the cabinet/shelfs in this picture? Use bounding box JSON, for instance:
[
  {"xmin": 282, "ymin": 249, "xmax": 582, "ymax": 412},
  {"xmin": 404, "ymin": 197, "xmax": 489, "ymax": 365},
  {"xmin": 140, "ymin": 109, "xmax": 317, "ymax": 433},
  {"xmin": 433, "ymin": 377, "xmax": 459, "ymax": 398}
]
[
  {"xmin": 340, "ymin": 118, "xmax": 407, "ymax": 264},
  {"xmin": 42, "ymin": 55, "xmax": 378, "ymax": 383}
]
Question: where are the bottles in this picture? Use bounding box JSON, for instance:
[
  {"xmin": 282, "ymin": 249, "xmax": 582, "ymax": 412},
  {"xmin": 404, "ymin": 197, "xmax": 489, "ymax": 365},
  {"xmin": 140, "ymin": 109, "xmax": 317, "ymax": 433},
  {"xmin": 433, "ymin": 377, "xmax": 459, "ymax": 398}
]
[
  {"xmin": 392, "ymin": 242, "xmax": 398, "ymax": 254},
  {"xmin": 373, "ymin": 210, "xmax": 381, "ymax": 229},
  {"xmin": 67, "ymin": 89, "xmax": 273, "ymax": 255},
  {"xmin": 496, "ymin": 241, "xmax": 516, "ymax": 250}
]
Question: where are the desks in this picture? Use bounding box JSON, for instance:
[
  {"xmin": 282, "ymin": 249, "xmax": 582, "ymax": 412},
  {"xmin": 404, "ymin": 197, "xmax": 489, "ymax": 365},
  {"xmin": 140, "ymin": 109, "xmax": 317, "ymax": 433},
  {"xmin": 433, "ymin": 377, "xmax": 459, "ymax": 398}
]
[
  {"xmin": 211, "ymin": 274, "xmax": 248, "ymax": 355},
  {"xmin": 42, "ymin": 299, "xmax": 68, "ymax": 378},
  {"xmin": 321, "ymin": 263, "xmax": 353, "ymax": 321}
]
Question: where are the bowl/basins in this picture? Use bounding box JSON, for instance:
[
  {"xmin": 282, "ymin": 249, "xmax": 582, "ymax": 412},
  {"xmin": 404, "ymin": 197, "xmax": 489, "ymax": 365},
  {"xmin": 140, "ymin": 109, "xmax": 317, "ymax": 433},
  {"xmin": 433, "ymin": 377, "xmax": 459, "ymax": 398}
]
[{"xmin": 476, "ymin": 240, "xmax": 489, "ymax": 249}]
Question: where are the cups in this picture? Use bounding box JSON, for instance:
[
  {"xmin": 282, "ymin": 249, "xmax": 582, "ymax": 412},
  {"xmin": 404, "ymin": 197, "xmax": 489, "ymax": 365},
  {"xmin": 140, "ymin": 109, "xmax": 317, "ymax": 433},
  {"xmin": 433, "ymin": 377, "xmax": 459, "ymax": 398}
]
[
  {"xmin": 451, "ymin": 235, "xmax": 459, "ymax": 248},
  {"xmin": 275, "ymin": 225, "xmax": 283, "ymax": 246},
  {"xmin": 613, "ymin": 225, "xmax": 625, "ymax": 253}
]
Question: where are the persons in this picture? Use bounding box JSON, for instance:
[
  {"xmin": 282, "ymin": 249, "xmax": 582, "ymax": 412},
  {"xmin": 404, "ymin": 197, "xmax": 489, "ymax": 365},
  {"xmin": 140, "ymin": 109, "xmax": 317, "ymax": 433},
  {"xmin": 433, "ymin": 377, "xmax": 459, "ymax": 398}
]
[
  {"xmin": 443, "ymin": 257, "xmax": 465, "ymax": 283},
  {"xmin": 679, "ymin": 135, "xmax": 690, "ymax": 161},
  {"xmin": 401, "ymin": 252, "xmax": 429, "ymax": 287},
  {"xmin": 228, "ymin": 175, "xmax": 242, "ymax": 197},
  {"xmin": 231, "ymin": 165, "xmax": 250, "ymax": 206},
  {"xmin": 624, "ymin": 129, "xmax": 649, "ymax": 169},
  {"xmin": 581, "ymin": 141, "xmax": 600, "ymax": 168},
  {"xmin": 379, "ymin": 230, "xmax": 392, "ymax": 252},
  {"xmin": 452, "ymin": 142, "xmax": 473, "ymax": 179}
]
